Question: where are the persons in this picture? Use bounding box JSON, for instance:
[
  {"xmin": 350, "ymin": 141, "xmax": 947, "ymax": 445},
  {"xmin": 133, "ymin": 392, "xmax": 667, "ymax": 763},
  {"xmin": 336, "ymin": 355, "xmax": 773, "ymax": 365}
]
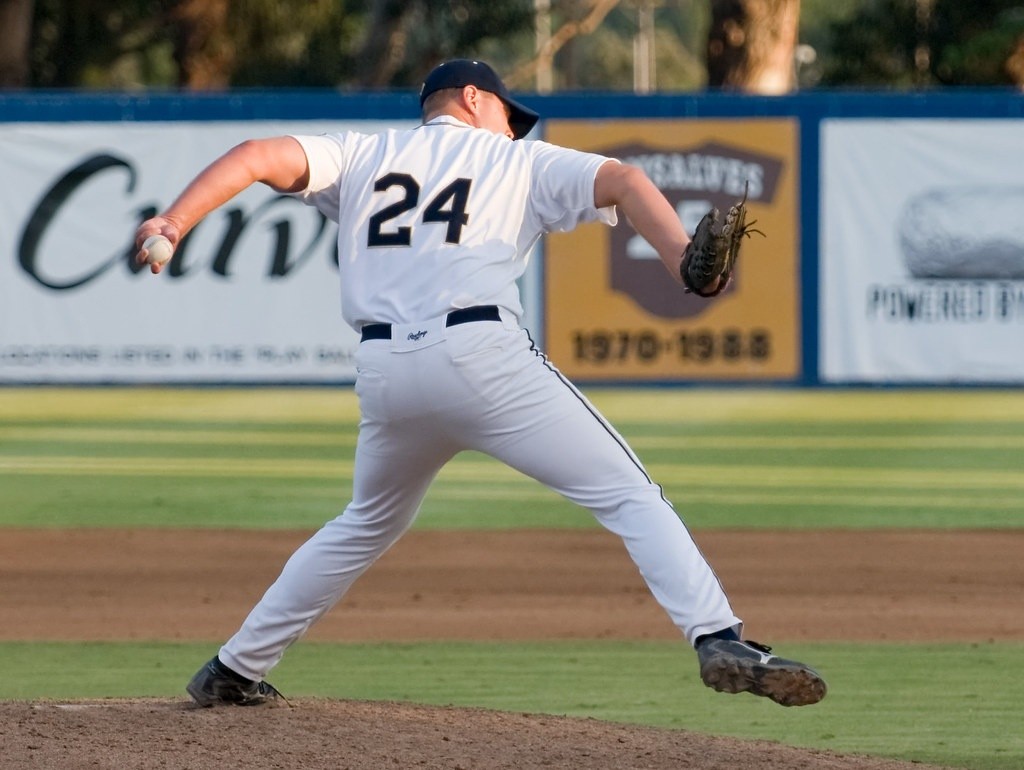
[{"xmin": 136, "ymin": 60, "xmax": 828, "ymax": 708}]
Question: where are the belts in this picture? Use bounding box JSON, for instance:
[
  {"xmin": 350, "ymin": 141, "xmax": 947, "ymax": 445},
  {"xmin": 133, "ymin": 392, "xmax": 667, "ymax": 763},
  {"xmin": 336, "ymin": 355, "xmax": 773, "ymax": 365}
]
[{"xmin": 360, "ymin": 306, "xmax": 502, "ymax": 342}]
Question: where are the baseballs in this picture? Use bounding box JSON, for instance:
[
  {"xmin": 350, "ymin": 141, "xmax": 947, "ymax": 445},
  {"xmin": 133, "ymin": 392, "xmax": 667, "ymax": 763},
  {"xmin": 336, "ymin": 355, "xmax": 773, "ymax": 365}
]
[{"xmin": 142, "ymin": 234, "xmax": 173, "ymax": 268}]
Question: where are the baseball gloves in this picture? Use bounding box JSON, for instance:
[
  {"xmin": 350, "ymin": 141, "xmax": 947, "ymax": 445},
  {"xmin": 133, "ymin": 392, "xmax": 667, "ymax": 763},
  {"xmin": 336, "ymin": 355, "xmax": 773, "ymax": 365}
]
[{"xmin": 679, "ymin": 201, "xmax": 746, "ymax": 300}]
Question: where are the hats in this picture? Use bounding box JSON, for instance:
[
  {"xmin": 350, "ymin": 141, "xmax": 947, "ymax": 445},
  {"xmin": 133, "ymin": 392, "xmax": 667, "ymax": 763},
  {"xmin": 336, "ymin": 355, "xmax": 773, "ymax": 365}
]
[{"xmin": 420, "ymin": 58, "xmax": 540, "ymax": 140}]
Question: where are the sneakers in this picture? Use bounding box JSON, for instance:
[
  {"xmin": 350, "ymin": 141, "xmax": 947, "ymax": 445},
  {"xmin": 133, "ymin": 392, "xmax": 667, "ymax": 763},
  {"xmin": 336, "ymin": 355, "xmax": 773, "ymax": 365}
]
[
  {"xmin": 187, "ymin": 656, "xmax": 291, "ymax": 708},
  {"xmin": 697, "ymin": 638, "xmax": 827, "ymax": 707}
]
